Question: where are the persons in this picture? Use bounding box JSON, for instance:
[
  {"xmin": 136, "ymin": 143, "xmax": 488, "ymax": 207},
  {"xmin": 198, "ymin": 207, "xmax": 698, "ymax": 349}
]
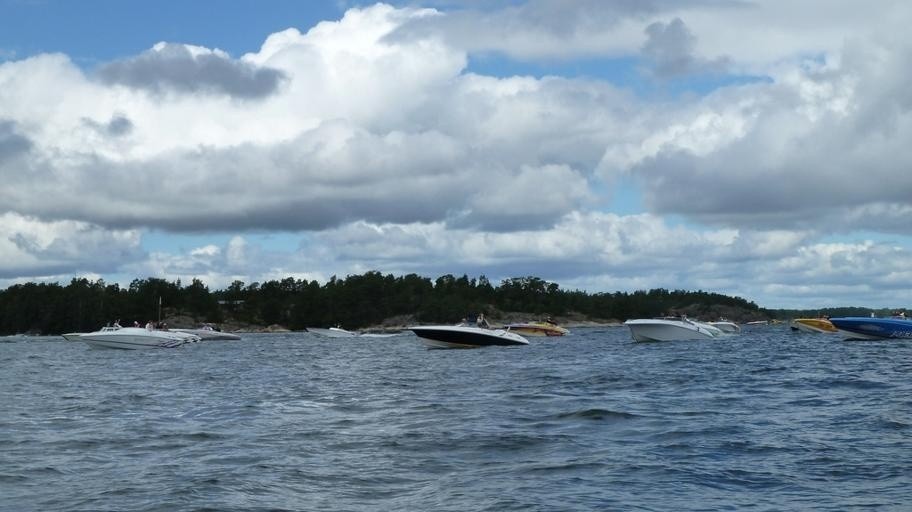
[
  {"xmin": 477, "ymin": 313, "xmax": 489, "ymax": 328},
  {"xmin": 892, "ymin": 310, "xmax": 905, "ymax": 319},
  {"xmin": 110, "ymin": 318, "xmax": 169, "ymax": 333},
  {"xmin": 547, "ymin": 312, "xmax": 552, "ymax": 321},
  {"xmin": 457, "ymin": 319, "xmax": 468, "ymax": 327}
]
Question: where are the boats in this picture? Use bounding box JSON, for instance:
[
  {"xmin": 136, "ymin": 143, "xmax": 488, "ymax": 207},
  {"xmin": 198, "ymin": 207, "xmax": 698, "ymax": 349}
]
[
  {"xmin": 503, "ymin": 318, "xmax": 571, "ymax": 338},
  {"xmin": 826, "ymin": 311, "xmax": 912, "ymax": 342},
  {"xmin": 61, "ymin": 333, "xmax": 91, "ymax": 343},
  {"xmin": 306, "ymin": 327, "xmax": 358, "ymax": 338},
  {"xmin": 624, "ymin": 315, "xmax": 741, "ymax": 345},
  {"xmin": 78, "ymin": 326, "xmax": 201, "ymax": 352},
  {"xmin": 408, "ymin": 321, "xmax": 530, "ymax": 349},
  {"xmin": 789, "ymin": 316, "xmax": 838, "ymax": 338},
  {"xmin": 768, "ymin": 318, "xmax": 784, "ymax": 328},
  {"xmin": 167, "ymin": 325, "xmax": 241, "ymax": 342}
]
